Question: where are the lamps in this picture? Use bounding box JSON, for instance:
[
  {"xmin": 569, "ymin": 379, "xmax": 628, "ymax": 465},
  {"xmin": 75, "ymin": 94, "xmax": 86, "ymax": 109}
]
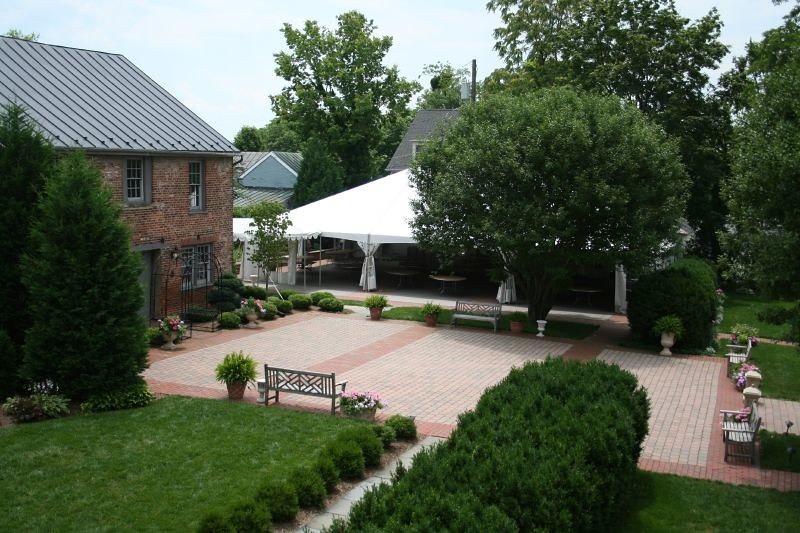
[
  {"xmin": 171, "ymin": 250, "xmax": 178, "ymax": 259},
  {"xmin": 785, "ymin": 420, "xmax": 793, "ymax": 428}
]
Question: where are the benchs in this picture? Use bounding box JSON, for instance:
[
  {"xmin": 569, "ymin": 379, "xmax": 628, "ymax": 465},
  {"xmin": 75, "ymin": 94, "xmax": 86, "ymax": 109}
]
[
  {"xmin": 724, "ymin": 339, "xmax": 752, "ymax": 377},
  {"xmin": 264, "ymin": 364, "xmax": 348, "ymax": 415},
  {"xmin": 720, "ymin": 399, "xmax": 761, "ymax": 465},
  {"xmin": 453, "ymin": 299, "xmax": 502, "ymax": 333}
]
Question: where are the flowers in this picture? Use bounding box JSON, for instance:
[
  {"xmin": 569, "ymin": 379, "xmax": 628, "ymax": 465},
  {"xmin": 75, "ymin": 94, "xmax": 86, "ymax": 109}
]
[
  {"xmin": 240, "ymin": 296, "xmax": 266, "ymax": 314},
  {"xmin": 719, "ymin": 406, "xmax": 752, "ymax": 427},
  {"xmin": 158, "ymin": 315, "xmax": 184, "ymax": 339},
  {"xmin": 339, "ymin": 389, "xmax": 383, "ymax": 415},
  {"xmin": 733, "ymin": 364, "xmax": 759, "ymax": 387}
]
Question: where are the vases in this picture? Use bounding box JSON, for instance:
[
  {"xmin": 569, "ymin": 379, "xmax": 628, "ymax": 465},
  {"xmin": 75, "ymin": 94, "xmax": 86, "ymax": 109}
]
[
  {"xmin": 246, "ymin": 314, "xmax": 258, "ymax": 329},
  {"xmin": 257, "ymin": 379, "xmax": 265, "ymax": 403},
  {"xmin": 536, "ymin": 320, "xmax": 547, "ymax": 337},
  {"xmin": 356, "ymin": 413, "xmax": 375, "ymax": 422},
  {"xmin": 161, "ymin": 331, "xmax": 177, "ymax": 350}
]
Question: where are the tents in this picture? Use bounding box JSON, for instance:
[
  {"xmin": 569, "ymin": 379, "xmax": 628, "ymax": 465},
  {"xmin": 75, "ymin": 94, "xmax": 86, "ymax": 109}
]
[
  {"xmin": 232, "ymin": 218, "xmax": 274, "ymax": 283},
  {"xmin": 248, "ymin": 165, "xmax": 687, "ymax": 317}
]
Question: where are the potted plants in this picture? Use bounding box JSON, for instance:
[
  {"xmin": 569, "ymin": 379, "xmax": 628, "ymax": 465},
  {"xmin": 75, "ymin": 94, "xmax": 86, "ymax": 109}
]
[
  {"xmin": 653, "ymin": 313, "xmax": 683, "ymax": 356},
  {"xmin": 364, "ymin": 293, "xmax": 387, "ymax": 320},
  {"xmin": 215, "ymin": 350, "xmax": 260, "ymax": 400},
  {"xmin": 421, "ymin": 303, "xmax": 440, "ymax": 326},
  {"xmin": 511, "ymin": 312, "xmax": 521, "ymax": 332}
]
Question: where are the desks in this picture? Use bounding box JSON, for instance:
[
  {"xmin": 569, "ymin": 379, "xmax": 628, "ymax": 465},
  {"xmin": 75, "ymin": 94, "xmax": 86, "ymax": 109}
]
[
  {"xmin": 569, "ymin": 287, "xmax": 600, "ymax": 306},
  {"xmin": 295, "ymin": 249, "xmax": 468, "ymax": 296}
]
